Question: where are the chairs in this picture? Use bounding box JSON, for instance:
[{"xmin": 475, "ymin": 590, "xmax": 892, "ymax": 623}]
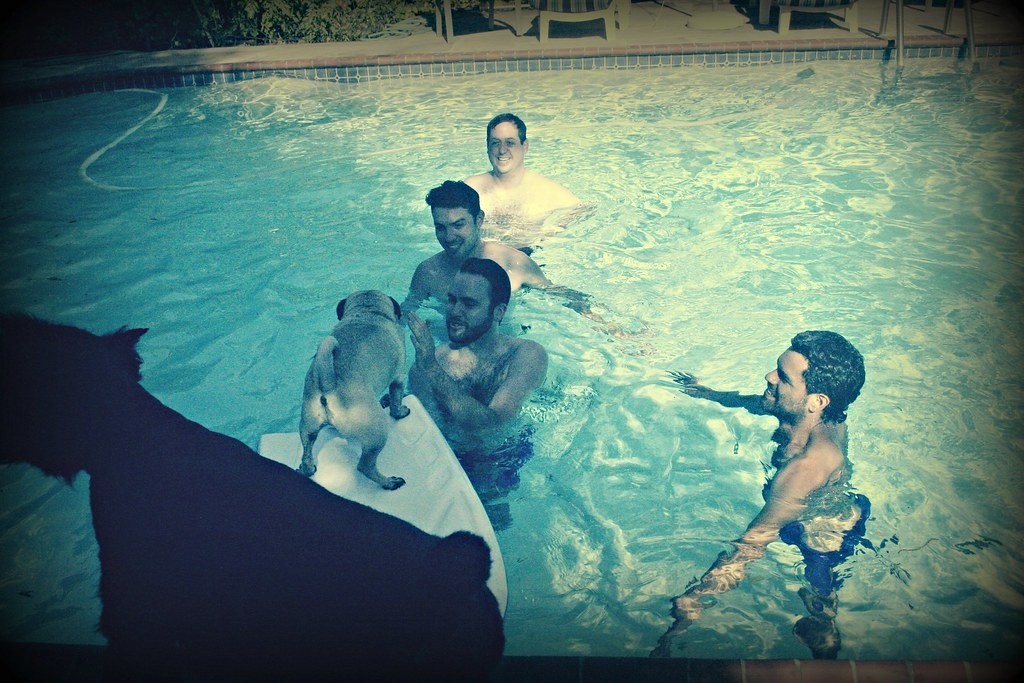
[
  {"xmin": 759, "ymin": 0, "xmax": 859, "ymax": 37},
  {"xmin": 539, "ymin": 0, "xmax": 629, "ymax": 45}
]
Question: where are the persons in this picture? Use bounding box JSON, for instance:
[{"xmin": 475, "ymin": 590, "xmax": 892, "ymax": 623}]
[
  {"xmin": 409, "ymin": 181, "xmax": 547, "ymax": 291},
  {"xmin": 462, "ymin": 113, "xmax": 571, "ymax": 202},
  {"xmin": 758, "ymin": 330, "xmax": 866, "ymax": 499},
  {"xmin": 407, "ymin": 258, "xmax": 549, "ymax": 426}
]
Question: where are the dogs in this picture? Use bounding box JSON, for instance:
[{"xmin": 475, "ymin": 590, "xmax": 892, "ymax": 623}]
[
  {"xmin": 295, "ymin": 290, "xmax": 411, "ymax": 490},
  {"xmin": 0, "ymin": 312, "xmax": 504, "ymax": 681}
]
